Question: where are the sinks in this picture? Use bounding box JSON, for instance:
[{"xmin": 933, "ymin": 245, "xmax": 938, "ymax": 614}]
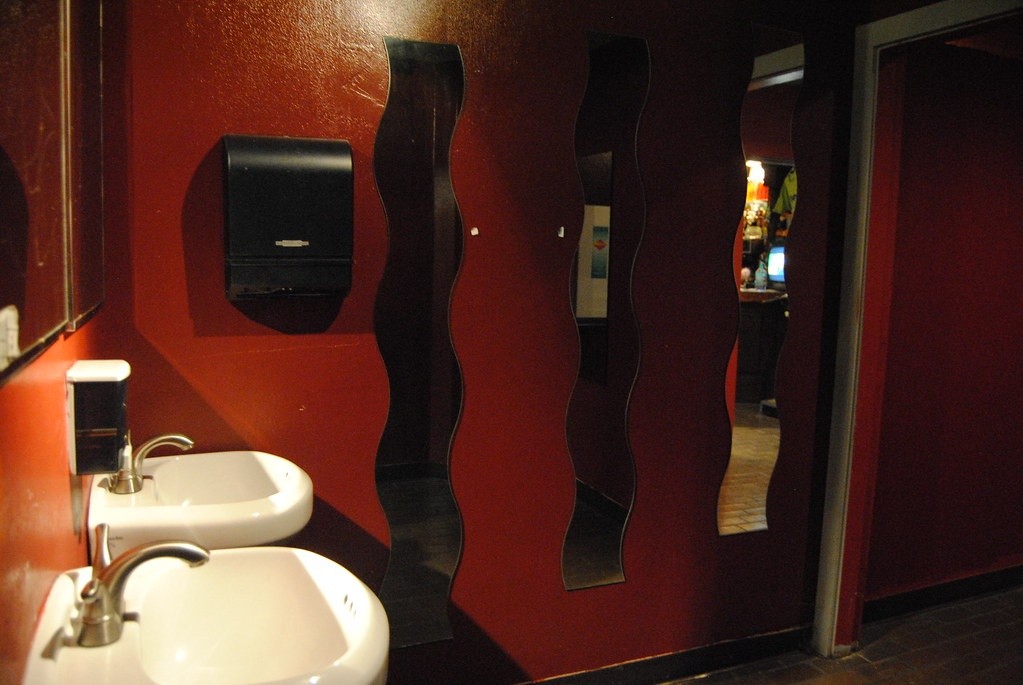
[
  {"xmin": 21, "ymin": 544, "xmax": 392, "ymax": 685},
  {"xmin": 86, "ymin": 450, "xmax": 316, "ymax": 567}
]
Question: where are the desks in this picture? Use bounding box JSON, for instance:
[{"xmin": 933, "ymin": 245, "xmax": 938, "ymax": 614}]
[{"xmin": 739, "ymin": 287, "xmax": 786, "ymax": 403}]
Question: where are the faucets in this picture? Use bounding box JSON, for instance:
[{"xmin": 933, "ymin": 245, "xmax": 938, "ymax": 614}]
[
  {"xmin": 97, "ymin": 538, "xmax": 211, "ymax": 624},
  {"xmin": 132, "ymin": 433, "xmax": 196, "ymax": 478}
]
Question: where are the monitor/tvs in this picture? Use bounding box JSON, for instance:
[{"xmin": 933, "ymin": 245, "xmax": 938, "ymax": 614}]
[{"xmin": 767, "ymin": 247, "xmax": 786, "ymax": 284}]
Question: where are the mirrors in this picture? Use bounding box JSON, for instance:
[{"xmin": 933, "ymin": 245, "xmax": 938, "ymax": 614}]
[{"xmin": 0, "ymin": 0, "xmax": 107, "ymax": 388}]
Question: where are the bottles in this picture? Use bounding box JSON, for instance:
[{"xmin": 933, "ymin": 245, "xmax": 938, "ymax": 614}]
[{"xmin": 754, "ymin": 252, "xmax": 768, "ymax": 289}]
